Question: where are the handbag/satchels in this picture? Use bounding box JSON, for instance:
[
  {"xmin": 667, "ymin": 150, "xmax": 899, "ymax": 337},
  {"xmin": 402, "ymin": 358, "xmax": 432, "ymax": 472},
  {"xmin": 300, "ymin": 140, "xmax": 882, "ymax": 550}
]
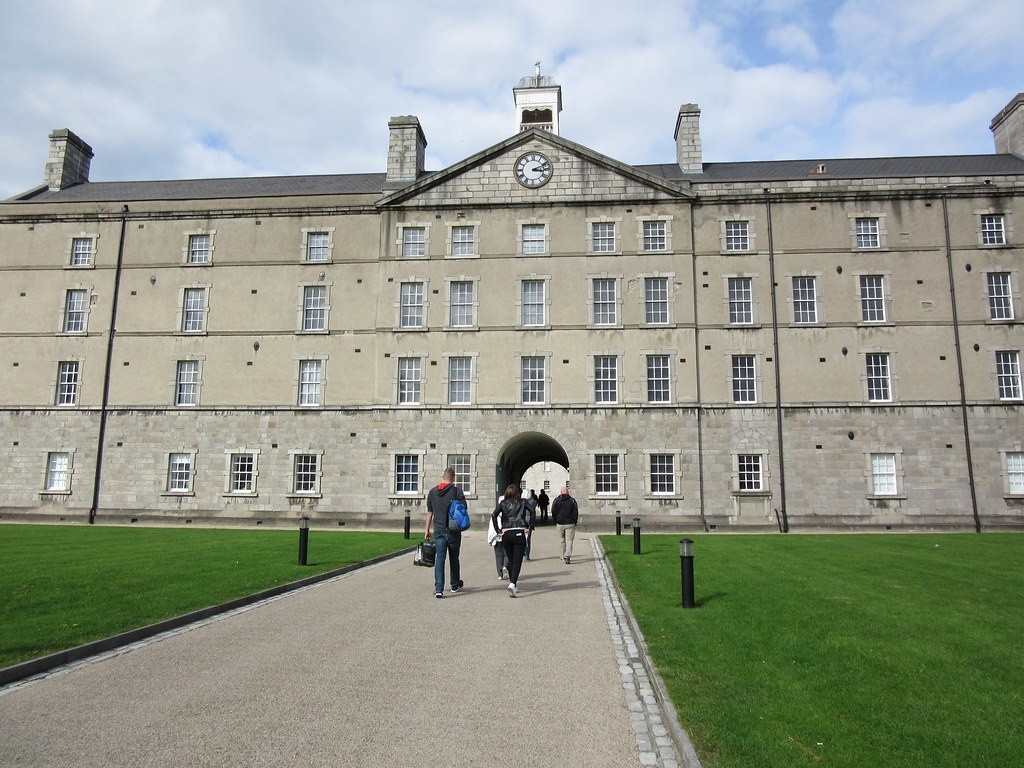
[{"xmin": 413, "ymin": 538, "xmax": 436, "ymax": 567}]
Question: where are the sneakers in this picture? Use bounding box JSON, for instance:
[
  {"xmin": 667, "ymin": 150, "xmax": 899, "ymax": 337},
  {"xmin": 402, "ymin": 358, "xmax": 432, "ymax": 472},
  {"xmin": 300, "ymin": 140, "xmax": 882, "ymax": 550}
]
[
  {"xmin": 451, "ymin": 580, "xmax": 464, "ymax": 592},
  {"xmin": 436, "ymin": 591, "xmax": 442, "ymax": 598}
]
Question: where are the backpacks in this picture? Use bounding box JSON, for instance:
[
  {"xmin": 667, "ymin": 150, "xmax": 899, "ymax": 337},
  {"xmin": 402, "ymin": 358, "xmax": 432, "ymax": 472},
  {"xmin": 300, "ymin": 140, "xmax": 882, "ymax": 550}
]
[{"xmin": 448, "ymin": 487, "xmax": 470, "ymax": 533}]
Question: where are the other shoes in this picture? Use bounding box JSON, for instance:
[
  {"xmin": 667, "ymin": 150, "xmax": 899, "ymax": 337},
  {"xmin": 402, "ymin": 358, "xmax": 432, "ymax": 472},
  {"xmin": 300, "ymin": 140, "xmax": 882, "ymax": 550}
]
[
  {"xmin": 503, "ymin": 568, "xmax": 508, "ymax": 581},
  {"xmin": 563, "ymin": 557, "xmax": 570, "ymax": 564},
  {"xmin": 508, "ymin": 582, "xmax": 519, "ymax": 598},
  {"xmin": 499, "ymin": 575, "xmax": 502, "ymax": 581}
]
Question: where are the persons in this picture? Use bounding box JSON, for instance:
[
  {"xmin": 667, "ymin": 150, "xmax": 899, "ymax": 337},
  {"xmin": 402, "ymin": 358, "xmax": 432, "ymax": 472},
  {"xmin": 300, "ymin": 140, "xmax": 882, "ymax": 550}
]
[
  {"xmin": 488, "ymin": 484, "xmax": 550, "ymax": 597},
  {"xmin": 551, "ymin": 487, "xmax": 578, "ymax": 564},
  {"xmin": 424, "ymin": 467, "xmax": 467, "ymax": 598}
]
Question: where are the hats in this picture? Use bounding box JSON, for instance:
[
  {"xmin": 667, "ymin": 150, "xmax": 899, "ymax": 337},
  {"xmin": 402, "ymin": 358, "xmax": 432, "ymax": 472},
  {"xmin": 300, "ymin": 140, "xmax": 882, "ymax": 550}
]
[
  {"xmin": 498, "ymin": 495, "xmax": 505, "ymax": 505},
  {"xmin": 561, "ymin": 487, "xmax": 568, "ymax": 495}
]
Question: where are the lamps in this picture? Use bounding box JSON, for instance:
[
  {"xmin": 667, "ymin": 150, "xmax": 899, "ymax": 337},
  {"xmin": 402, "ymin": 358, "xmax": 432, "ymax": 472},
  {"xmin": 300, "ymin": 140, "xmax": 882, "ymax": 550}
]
[
  {"xmin": 300, "ymin": 517, "xmax": 310, "ymax": 528},
  {"xmin": 633, "ymin": 517, "xmax": 641, "ymax": 527},
  {"xmin": 616, "ymin": 510, "xmax": 621, "ymax": 518},
  {"xmin": 405, "ymin": 510, "xmax": 410, "ymax": 517},
  {"xmin": 679, "ymin": 538, "xmax": 694, "ymax": 556}
]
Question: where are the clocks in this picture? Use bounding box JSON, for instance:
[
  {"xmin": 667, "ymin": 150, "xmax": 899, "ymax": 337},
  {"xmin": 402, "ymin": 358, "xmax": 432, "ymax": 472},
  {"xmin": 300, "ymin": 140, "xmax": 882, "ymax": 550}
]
[{"xmin": 513, "ymin": 151, "xmax": 553, "ymax": 189}]
